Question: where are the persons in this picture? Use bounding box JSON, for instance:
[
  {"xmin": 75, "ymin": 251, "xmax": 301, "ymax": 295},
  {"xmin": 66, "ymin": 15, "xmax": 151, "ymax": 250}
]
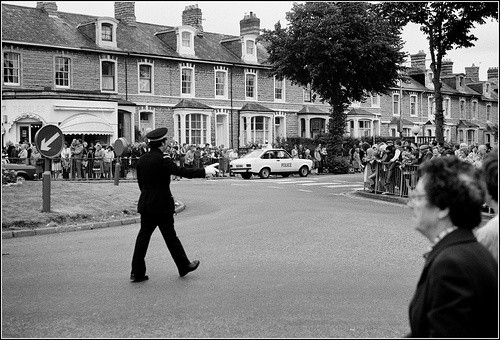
[
  {"xmin": 130, "ymin": 127, "xmax": 200, "ymax": 283},
  {"xmin": 404, "ymin": 156, "xmax": 498, "ymax": 338},
  {"xmin": 356, "ymin": 141, "xmax": 492, "ymax": 202},
  {"xmin": 2, "ymin": 138, "xmax": 363, "ymax": 181},
  {"xmin": 473, "ymin": 146, "xmax": 498, "ymax": 261}
]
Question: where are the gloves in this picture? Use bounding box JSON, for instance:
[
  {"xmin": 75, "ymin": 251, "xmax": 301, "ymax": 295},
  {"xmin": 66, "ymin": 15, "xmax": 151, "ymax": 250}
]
[{"xmin": 204, "ymin": 163, "xmax": 219, "ymax": 176}]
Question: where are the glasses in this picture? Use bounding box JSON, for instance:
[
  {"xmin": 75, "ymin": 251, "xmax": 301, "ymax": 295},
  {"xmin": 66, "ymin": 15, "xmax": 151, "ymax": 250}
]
[{"xmin": 408, "ymin": 190, "xmax": 427, "ymax": 205}]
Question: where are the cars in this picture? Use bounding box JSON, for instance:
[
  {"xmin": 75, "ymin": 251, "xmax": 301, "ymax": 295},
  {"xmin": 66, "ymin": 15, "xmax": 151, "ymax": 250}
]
[
  {"xmin": 2, "ymin": 159, "xmax": 37, "ymax": 183},
  {"xmin": 229, "ymin": 148, "xmax": 314, "ymax": 179}
]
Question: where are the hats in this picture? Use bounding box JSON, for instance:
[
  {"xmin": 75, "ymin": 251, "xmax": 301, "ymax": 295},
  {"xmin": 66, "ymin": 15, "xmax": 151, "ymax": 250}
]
[{"xmin": 146, "ymin": 127, "xmax": 168, "ymax": 141}]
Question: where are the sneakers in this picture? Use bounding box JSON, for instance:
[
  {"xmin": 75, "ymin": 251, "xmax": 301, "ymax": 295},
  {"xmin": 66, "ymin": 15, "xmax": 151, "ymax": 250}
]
[{"xmin": 382, "ymin": 191, "xmax": 389, "ymax": 195}]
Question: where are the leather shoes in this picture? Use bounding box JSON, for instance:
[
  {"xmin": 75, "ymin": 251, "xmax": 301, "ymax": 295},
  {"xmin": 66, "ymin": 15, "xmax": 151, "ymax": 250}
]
[
  {"xmin": 179, "ymin": 260, "xmax": 200, "ymax": 276},
  {"xmin": 130, "ymin": 274, "xmax": 149, "ymax": 282}
]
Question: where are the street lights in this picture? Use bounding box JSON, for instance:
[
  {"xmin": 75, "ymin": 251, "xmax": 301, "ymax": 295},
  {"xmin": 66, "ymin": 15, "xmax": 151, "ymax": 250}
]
[{"xmin": 412, "ymin": 124, "xmax": 421, "ymax": 144}]
